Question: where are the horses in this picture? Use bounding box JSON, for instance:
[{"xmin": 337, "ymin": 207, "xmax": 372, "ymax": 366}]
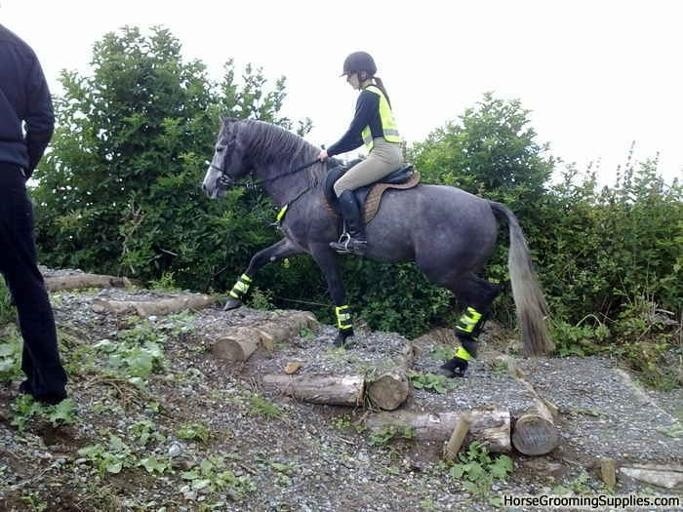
[{"xmin": 203, "ymin": 112, "xmax": 559, "ymax": 379}]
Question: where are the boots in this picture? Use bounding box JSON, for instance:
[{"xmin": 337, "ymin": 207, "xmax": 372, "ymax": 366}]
[{"xmin": 329, "ymin": 190, "xmax": 367, "ymax": 256}]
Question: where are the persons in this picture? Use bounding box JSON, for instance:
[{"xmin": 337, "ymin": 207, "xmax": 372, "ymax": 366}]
[
  {"xmin": 2, "ymin": 19, "xmax": 65, "ymax": 402},
  {"xmin": 318, "ymin": 50, "xmax": 404, "ymax": 258}
]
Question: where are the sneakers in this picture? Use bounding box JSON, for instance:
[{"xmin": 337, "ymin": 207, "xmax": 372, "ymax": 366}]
[{"xmin": 20, "ymin": 380, "xmax": 67, "ymax": 404}]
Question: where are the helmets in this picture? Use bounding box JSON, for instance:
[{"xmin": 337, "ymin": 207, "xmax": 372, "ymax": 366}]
[{"xmin": 340, "ymin": 52, "xmax": 376, "ymax": 78}]
